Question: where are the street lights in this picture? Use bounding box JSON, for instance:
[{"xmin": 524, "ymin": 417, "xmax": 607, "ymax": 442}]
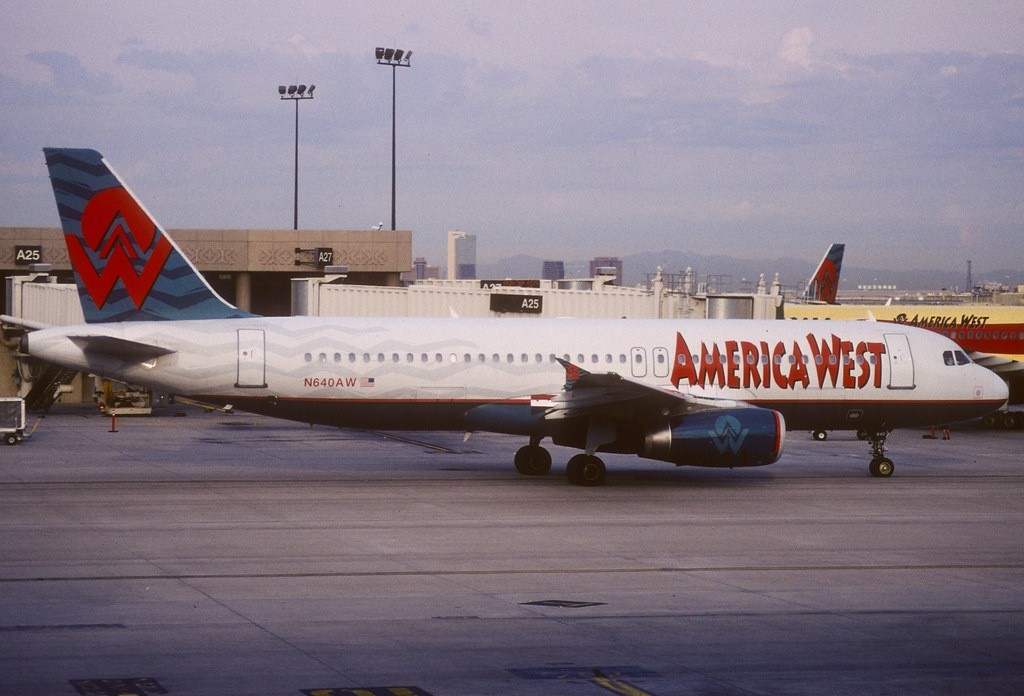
[
  {"xmin": 276, "ymin": 85, "xmax": 320, "ymax": 229},
  {"xmin": 372, "ymin": 47, "xmax": 414, "ymax": 231}
]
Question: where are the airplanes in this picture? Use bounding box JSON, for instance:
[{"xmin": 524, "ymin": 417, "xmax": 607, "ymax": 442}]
[
  {"xmin": 0, "ymin": 146, "xmax": 1011, "ymax": 485},
  {"xmin": 777, "ymin": 237, "xmax": 1024, "ymax": 437}
]
